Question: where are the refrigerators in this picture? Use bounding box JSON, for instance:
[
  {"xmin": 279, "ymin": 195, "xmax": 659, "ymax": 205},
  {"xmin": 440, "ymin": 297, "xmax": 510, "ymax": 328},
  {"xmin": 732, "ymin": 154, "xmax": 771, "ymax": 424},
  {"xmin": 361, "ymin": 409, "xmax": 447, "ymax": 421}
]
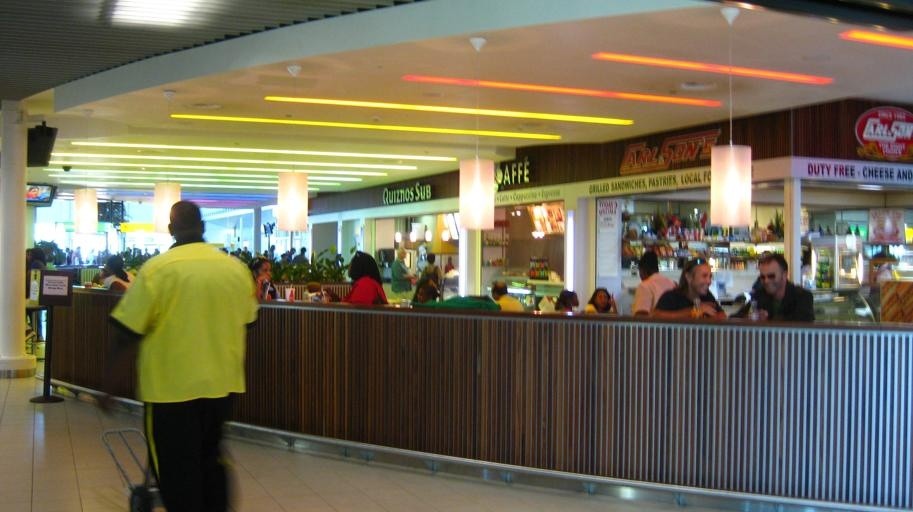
[{"xmin": 808, "ymin": 235, "xmax": 864, "ymax": 291}]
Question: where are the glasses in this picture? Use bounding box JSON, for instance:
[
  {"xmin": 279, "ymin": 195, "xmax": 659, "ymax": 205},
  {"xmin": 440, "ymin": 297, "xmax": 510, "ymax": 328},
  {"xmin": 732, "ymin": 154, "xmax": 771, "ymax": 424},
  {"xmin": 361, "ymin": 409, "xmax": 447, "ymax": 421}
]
[{"xmin": 758, "ymin": 273, "xmax": 779, "ymax": 280}]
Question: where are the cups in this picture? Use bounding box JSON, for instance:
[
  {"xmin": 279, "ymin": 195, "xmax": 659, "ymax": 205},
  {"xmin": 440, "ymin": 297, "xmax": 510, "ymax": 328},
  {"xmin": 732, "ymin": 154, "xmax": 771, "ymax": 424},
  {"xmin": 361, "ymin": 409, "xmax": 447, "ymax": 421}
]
[{"xmin": 285, "ymin": 287, "xmax": 296, "ymax": 303}]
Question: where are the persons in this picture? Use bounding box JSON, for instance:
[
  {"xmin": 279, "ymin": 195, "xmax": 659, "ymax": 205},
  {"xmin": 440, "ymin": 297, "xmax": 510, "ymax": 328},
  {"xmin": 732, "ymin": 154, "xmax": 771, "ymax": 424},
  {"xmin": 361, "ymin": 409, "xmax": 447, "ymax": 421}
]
[
  {"xmin": 632, "ymin": 252, "xmax": 728, "ymax": 325},
  {"xmin": 91, "ymin": 255, "xmax": 137, "ymax": 290},
  {"xmin": 491, "ymin": 281, "xmax": 526, "ymax": 312},
  {"xmin": 555, "ymin": 287, "xmax": 617, "ymax": 316},
  {"xmin": 746, "ymin": 251, "xmax": 817, "ymax": 326},
  {"xmin": 25, "ymin": 187, "xmax": 39, "ymax": 198},
  {"xmin": 390, "ymin": 245, "xmax": 455, "ymax": 307},
  {"xmin": 65, "ymin": 245, "xmax": 83, "ymax": 264},
  {"xmin": 248, "ymin": 258, "xmax": 280, "ymax": 300},
  {"xmin": 121, "ymin": 247, "xmax": 160, "ymax": 264},
  {"xmin": 106, "ymin": 197, "xmax": 262, "ymax": 511},
  {"xmin": 219, "ymin": 245, "xmax": 309, "ymax": 267},
  {"xmin": 323, "ymin": 251, "xmax": 387, "ymax": 306},
  {"xmin": 27, "ymin": 244, "xmax": 47, "ymax": 267}
]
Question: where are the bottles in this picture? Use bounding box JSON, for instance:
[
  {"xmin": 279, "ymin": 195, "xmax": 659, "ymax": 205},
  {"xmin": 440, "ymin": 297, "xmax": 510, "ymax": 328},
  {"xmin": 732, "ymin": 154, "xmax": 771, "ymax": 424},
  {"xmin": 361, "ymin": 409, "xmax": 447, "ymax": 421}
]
[
  {"xmin": 691, "ymin": 298, "xmax": 704, "ymax": 321},
  {"xmin": 748, "ymin": 301, "xmax": 760, "ymax": 321}
]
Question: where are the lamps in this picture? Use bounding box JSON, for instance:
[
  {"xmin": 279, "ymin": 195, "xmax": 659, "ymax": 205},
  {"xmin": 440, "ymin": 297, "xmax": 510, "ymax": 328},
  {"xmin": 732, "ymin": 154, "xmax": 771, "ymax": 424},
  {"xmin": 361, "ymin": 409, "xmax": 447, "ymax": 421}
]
[
  {"xmin": 277, "ymin": 62, "xmax": 309, "ymax": 234},
  {"xmin": 73, "ymin": 108, "xmax": 99, "ymax": 235},
  {"xmin": 459, "ymin": 36, "xmax": 496, "ymax": 231},
  {"xmin": 710, "ymin": 5, "xmax": 751, "ymax": 229},
  {"xmin": 155, "ymin": 87, "xmax": 180, "ymax": 232}
]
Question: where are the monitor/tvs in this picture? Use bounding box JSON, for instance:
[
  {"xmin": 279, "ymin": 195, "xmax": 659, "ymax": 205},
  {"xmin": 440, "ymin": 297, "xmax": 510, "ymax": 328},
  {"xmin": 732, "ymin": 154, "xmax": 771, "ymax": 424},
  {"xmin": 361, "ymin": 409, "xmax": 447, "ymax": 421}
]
[{"xmin": 26, "ymin": 184, "xmax": 53, "ymax": 203}]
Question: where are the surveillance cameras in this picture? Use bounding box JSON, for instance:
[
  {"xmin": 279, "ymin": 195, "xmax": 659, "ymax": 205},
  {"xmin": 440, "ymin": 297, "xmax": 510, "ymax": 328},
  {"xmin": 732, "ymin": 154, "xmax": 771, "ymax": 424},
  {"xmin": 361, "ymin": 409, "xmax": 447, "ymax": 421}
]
[{"xmin": 63, "ymin": 166, "xmax": 71, "ymax": 171}]
